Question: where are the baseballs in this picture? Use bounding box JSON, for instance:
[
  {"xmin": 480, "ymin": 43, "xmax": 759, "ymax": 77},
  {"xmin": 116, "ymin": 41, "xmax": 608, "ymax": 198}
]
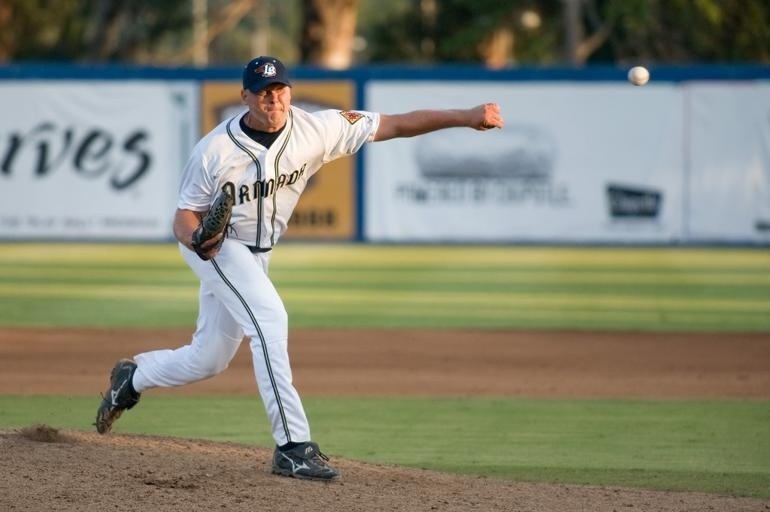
[{"xmin": 627, "ymin": 65, "xmax": 650, "ymax": 87}]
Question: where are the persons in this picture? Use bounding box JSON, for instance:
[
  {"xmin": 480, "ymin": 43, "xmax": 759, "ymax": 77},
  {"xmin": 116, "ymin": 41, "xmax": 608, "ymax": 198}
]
[{"xmin": 91, "ymin": 55, "xmax": 504, "ymax": 482}]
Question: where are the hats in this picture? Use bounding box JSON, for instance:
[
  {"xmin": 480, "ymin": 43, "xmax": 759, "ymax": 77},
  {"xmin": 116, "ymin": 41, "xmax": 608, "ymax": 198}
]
[{"xmin": 241, "ymin": 55, "xmax": 293, "ymax": 95}]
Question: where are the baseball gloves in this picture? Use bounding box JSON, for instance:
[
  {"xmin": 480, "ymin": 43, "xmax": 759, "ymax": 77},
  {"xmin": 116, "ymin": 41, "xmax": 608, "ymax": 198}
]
[{"xmin": 190, "ymin": 191, "xmax": 238, "ymax": 261}]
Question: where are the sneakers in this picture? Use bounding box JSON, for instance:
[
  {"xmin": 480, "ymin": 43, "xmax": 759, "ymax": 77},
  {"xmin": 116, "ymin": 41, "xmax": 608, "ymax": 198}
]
[
  {"xmin": 270, "ymin": 441, "xmax": 343, "ymax": 483},
  {"xmin": 93, "ymin": 359, "xmax": 143, "ymax": 435}
]
